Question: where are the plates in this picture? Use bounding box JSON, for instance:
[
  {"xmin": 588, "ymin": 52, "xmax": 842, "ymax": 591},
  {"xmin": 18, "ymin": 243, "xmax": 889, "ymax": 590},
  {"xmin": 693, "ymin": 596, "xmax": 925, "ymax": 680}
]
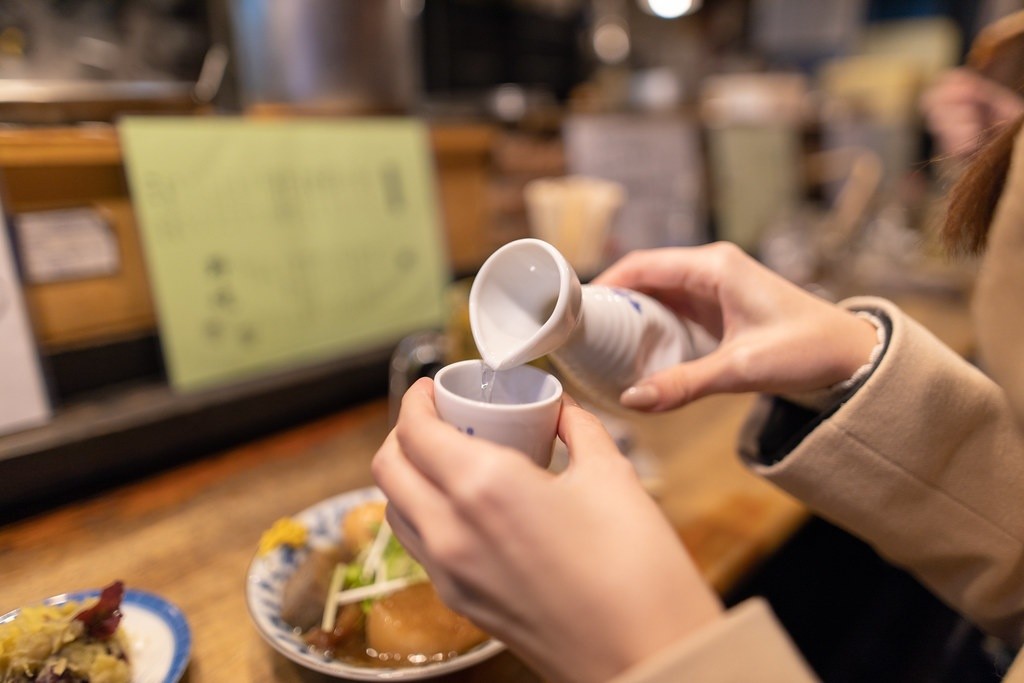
[
  {"xmin": 0, "ymin": 589, "xmax": 191, "ymax": 682},
  {"xmin": 243, "ymin": 486, "xmax": 509, "ymax": 683}
]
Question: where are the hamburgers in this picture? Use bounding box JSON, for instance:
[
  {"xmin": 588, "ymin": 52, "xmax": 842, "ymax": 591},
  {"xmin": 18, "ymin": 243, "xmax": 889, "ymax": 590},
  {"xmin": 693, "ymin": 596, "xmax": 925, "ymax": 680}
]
[{"xmin": 319, "ymin": 490, "xmax": 495, "ymax": 668}]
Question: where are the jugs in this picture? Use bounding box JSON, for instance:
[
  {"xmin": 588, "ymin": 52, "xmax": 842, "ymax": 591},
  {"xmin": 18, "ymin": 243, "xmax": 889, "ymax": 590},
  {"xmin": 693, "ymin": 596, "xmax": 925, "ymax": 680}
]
[{"xmin": 389, "ymin": 281, "xmax": 563, "ymax": 430}]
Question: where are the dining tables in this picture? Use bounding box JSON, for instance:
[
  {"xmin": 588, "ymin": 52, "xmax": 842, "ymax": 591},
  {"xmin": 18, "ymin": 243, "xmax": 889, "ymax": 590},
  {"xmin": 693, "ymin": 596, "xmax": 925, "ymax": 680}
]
[{"xmin": 0, "ymin": 287, "xmax": 981, "ymax": 683}]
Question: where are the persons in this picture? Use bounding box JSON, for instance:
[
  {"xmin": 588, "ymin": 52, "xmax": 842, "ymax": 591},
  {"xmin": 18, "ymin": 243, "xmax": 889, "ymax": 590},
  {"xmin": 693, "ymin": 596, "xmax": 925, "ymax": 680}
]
[{"xmin": 372, "ymin": 14, "xmax": 1024, "ymax": 683}]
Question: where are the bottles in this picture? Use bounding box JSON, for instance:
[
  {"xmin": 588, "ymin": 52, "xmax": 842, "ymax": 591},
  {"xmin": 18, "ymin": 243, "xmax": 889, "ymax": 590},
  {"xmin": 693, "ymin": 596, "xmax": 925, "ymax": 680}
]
[{"xmin": 468, "ymin": 237, "xmax": 700, "ymax": 421}]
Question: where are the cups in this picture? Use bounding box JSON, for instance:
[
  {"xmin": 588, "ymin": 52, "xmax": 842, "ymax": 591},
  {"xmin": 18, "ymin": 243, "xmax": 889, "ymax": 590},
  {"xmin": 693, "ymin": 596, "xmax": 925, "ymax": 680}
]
[
  {"xmin": 435, "ymin": 359, "xmax": 564, "ymax": 472},
  {"xmin": 524, "ymin": 177, "xmax": 627, "ymax": 277}
]
